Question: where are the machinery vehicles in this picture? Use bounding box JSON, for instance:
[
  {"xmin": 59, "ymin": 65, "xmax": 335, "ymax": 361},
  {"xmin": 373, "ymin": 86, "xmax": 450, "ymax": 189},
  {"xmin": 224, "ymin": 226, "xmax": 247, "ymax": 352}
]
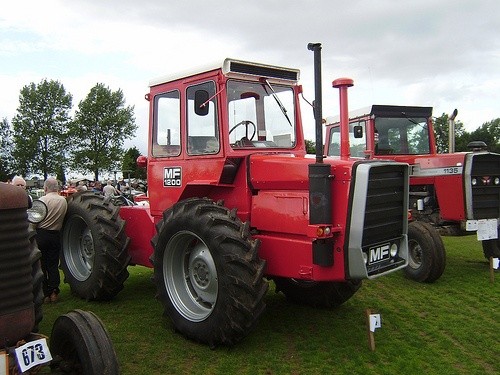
[
  {"xmin": 58, "ymin": 43, "xmax": 410, "ymax": 350},
  {"xmin": 324, "ymin": 104, "xmax": 500, "ymax": 284},
  {"xmin": 0, "ymin": 181, "xmax": 119, "ymax": 375}
]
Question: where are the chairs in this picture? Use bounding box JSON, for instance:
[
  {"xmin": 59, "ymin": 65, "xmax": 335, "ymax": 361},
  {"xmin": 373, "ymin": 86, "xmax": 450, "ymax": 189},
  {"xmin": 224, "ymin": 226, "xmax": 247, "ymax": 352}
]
[{"xmin": 187, "ymin": 135, "xmax": 218, "ymax": 155}]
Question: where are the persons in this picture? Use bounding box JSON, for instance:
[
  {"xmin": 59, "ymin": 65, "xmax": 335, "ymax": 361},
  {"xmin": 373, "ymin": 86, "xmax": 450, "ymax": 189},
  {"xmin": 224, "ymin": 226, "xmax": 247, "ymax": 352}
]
[
  {"xmin": 31, "ymin": 177, "xmax": 67, "ymax": 303},
  {"xmin": 12, "ymin": 174, "xmax": 148, "ymax": 216}
]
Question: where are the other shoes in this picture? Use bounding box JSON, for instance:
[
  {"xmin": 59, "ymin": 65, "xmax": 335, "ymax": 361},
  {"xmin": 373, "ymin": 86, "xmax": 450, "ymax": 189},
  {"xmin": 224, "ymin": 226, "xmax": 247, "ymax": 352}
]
[{"xmin": 43, "ymin": 292, "xmax": 57, "ymax": 304}]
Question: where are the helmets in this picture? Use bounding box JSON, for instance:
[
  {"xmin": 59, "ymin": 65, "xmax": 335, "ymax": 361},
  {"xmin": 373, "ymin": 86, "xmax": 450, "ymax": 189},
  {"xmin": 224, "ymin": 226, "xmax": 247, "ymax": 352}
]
[{"xmin": 137, "ymin": 156, "xmax": 148, "ymax": 169}]
